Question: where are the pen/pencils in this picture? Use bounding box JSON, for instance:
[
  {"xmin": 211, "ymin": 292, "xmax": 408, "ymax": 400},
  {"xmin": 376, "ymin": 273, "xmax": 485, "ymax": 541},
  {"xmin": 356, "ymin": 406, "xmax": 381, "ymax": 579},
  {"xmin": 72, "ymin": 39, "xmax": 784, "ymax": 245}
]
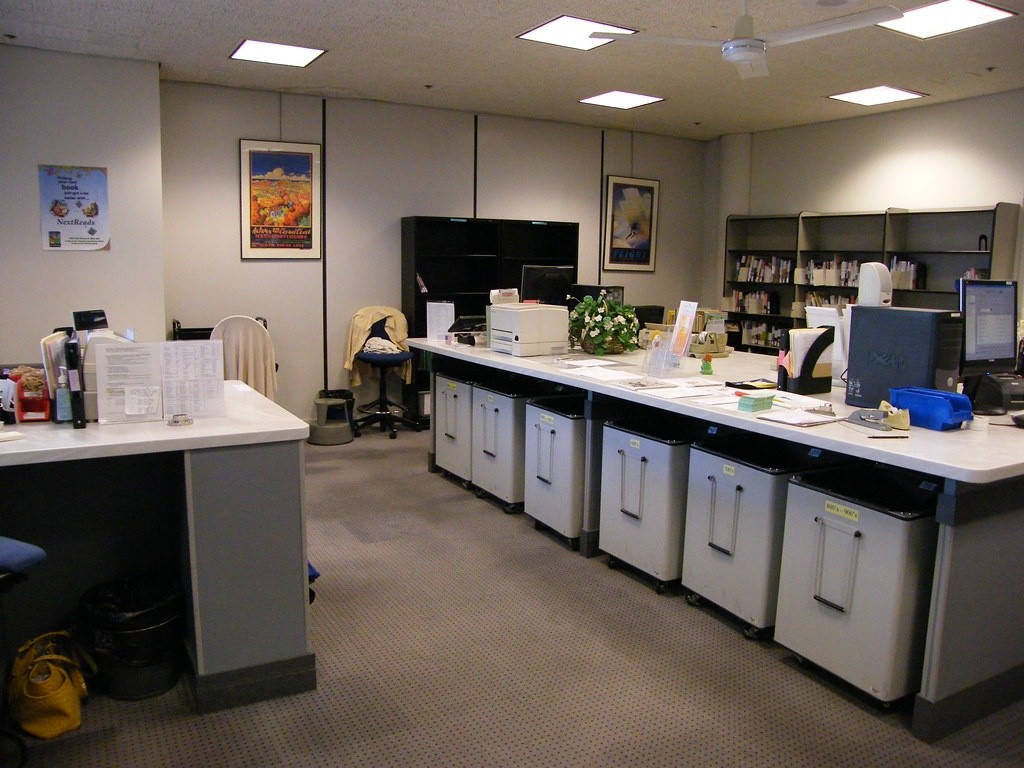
[{"xmin": 868, "ymin": 436, "xmax": 909, "ymax": 438}]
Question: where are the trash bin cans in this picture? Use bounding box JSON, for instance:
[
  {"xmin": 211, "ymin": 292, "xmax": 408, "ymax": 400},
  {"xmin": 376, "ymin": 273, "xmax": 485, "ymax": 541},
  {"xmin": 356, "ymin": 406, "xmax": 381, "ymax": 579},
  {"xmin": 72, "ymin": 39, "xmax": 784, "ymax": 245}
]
[
  {"xmin": 79, "ymin": 570, "xmax": 186, "ymax": 699},
  {"xmin": 318, "ymin": 389, "xmax": 354, "ymax": 421}
]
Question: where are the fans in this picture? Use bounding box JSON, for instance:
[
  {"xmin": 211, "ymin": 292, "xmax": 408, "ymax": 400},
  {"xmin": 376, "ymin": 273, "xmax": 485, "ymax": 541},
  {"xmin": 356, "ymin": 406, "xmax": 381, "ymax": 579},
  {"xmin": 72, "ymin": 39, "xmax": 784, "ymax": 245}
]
[{"xmin": 589, "ymin": 0, "xmax": 904, "ymax": 80}]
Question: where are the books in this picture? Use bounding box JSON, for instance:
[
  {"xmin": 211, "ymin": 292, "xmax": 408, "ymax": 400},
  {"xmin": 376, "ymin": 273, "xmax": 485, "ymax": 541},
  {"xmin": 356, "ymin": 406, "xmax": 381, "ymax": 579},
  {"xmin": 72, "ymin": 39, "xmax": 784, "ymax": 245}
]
[
  {"xmin": 803, "ymin": 291, "xmax": 859, "ymax": 316},
  {"xmin": 889, "ymin": 255, "xmax": 927, "ymax": 291},
  {"xmin": 733, "ymin": 253, "xmax": 796, "ymax": 284},
  {"xmin": 741, "ymin": 321, "xmax": 790, "ymax": 349},
  {"xmin": 963, "ymin": 267, "xmax": 991, "ymax": 293},
  {"xmin": 731, "ymin": 288, "xmax": 781, "ymax": 316},
  {"xmin": 673, "ymin": 307, "xmax": 725, "ymax": 335},
  {"xmin": 788, "ymin": 327, "xmax": 832, "ymax": 380},
  {"xmin": 802, "ymin": 253, "xmax": 875, "ymax": 288},
  {"xmin": 40, "ymin": 331, "xmax": 70, "ymax": 399}
]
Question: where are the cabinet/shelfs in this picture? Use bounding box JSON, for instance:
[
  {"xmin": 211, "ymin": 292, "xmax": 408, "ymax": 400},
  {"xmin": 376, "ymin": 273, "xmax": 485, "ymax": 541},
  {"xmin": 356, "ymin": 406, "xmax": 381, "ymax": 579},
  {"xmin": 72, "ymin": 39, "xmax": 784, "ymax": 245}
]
[
  {"xmin": 171, "ymin": 316, "xmax": 266, "ymax": 341},
  {"xmin": 401, "ymin": 215, "xmax": 580, "ymax": 432},
  {"xmin": 720, "ymin": 202, "xmax": 1024, "ymax": 356}
]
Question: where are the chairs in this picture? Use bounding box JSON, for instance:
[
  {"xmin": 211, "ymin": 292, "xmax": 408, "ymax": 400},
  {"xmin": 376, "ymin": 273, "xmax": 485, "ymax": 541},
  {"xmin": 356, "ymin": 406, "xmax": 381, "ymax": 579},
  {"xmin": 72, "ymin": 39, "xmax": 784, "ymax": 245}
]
[{"xmin": 351, "ymin": 304, "xmax": 422, "ymax": 439}]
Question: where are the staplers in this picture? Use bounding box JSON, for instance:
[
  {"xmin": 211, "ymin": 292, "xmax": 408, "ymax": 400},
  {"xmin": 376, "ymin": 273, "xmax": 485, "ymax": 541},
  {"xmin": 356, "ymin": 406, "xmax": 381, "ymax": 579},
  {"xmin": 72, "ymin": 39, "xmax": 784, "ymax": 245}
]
[{"xmin": 847, "ymin": 408, "xmax": 893, "ymax": 431}]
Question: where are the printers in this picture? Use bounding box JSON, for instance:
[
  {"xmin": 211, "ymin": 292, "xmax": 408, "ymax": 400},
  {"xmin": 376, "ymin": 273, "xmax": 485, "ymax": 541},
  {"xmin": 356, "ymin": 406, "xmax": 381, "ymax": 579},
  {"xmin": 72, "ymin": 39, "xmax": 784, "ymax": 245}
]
[{"xmin": 490, "ymin": 303, "xmax": 569, "ymax": 357}]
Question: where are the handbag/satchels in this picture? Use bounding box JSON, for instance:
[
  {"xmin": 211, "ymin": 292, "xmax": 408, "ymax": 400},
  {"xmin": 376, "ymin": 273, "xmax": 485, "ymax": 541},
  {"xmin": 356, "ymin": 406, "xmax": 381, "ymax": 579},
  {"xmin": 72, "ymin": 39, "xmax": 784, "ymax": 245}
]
[{"xmin": 7, "ymin": 631, "xmax": 88, "ymax": 739}]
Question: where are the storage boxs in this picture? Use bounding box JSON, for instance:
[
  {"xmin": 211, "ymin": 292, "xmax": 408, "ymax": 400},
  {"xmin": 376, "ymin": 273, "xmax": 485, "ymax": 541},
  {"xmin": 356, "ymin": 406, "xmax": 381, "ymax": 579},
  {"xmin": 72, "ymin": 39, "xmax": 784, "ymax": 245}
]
[
  {"xmin": 681, "ymin": 424, "xmax": 846, "ymax": 640},
  {"xmin": 471, "ymin": 377, "xmax": 574, "ymax": 514},
  {"xmin": 524, "ymin": 391, "xmax": 650, "ymax": 552},
  {"xmin": 435, "ymin": 367, "xmax": 519, "ymax": 488},
  {"xmin": 599, "ymin": 407, "xmax": 735, "ymax": 596},
  {"xmin": 774, "ymin": 454, "xmax": 947, "ymax": 707}
]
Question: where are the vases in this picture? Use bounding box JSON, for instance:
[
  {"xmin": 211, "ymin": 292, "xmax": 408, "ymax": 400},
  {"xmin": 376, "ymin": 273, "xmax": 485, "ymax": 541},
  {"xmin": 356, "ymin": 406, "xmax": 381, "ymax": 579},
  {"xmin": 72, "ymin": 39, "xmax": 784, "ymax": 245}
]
[{"xmin": 577, "ymin": 325, "xmax": 625, "ymax": 354}]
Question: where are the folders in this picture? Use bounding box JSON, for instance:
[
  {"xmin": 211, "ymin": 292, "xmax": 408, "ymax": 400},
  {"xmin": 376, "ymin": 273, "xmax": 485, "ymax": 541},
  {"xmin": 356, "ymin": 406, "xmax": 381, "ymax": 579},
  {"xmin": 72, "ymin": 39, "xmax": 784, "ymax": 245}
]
[{"xmin": 64, "ymin": 332, "xmax": 86, "ymax": 429}]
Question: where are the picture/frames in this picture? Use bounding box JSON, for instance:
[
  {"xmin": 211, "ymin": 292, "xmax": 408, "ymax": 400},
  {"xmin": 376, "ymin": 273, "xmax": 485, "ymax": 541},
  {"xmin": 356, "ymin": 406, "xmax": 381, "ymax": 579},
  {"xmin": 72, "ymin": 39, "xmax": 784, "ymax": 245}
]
[
  {"xmin": 239, "ymin": 138, "xmax": 322, "ymax": 260},
  {"xmin": 603, "ymin": 175, "xmax": 660, "ymax": 271}
]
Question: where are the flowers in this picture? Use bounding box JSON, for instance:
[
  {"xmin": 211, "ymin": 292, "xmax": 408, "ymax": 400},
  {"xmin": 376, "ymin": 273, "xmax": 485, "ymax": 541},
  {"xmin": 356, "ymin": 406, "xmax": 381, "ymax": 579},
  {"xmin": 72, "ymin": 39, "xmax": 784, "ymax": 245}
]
[{"xmin": 565, "ymin": 289, "xmax": 640, "ymax": 355}]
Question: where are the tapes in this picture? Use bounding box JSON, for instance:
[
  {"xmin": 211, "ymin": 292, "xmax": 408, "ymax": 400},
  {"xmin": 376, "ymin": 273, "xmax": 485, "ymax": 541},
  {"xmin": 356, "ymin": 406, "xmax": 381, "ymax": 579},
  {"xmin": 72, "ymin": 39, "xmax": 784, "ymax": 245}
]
[
  {"xmin": 699, "ymin": 331, "xmax": 708, "ymax": 342},
  {"xmin": 974, "ymin": 415, "xmax": 989, "ymax": 426},
  {"xmin": 888, "ymin": 407, "xmax": 898, "ymax": 415}
]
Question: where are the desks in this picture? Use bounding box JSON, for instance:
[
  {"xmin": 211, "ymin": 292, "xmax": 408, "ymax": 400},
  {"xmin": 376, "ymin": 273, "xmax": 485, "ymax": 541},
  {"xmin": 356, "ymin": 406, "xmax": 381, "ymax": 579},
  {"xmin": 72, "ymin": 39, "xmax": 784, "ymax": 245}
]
[
  {"xmin": 0, "ymin": 380, "xmax": 315, "ymax": 715},
  {"xmin": 405, "ymin": 334, "xmax": 1024, "ymax": 743}
]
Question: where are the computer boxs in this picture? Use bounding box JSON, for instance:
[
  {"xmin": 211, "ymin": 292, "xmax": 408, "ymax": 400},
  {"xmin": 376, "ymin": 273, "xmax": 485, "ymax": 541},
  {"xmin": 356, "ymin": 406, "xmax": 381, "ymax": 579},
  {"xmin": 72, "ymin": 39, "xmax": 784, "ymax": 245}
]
[
  {"xmin": 570, "ymin": 283, "xmax": 624, "ymax": 311},
  {"xmin": 843, "ymin": 306, "xmax": 964, "ymax": 409}
]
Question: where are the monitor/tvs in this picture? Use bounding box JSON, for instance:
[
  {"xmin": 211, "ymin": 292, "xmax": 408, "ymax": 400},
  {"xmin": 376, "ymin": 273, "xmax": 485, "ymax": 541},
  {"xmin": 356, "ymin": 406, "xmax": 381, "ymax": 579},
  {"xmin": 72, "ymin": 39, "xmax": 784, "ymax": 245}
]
[
  {"xmin": 521, "ymin": 265, "xmax": 576, "ymax": 307},
  {"xmin": 959, "ymin": 277, "xmax": 1018, "ymax": 416}
]
